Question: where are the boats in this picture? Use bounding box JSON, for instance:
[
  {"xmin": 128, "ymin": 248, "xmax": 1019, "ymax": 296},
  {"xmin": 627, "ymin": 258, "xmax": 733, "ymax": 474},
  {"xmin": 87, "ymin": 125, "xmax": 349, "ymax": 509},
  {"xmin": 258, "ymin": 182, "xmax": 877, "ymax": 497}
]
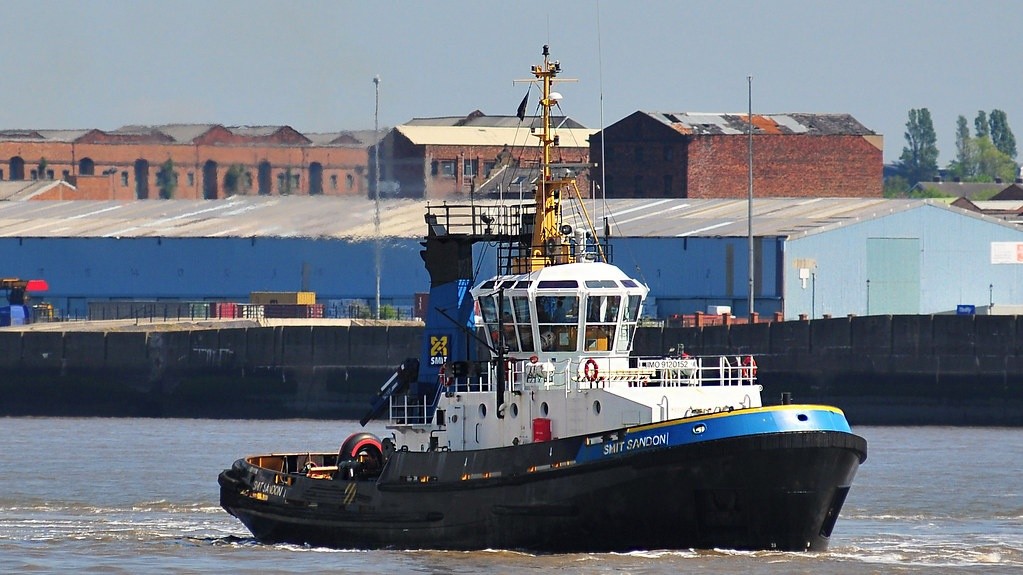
[{"xmin": 218, "ymin": 0, "xmax": 867, "ymax": 552}]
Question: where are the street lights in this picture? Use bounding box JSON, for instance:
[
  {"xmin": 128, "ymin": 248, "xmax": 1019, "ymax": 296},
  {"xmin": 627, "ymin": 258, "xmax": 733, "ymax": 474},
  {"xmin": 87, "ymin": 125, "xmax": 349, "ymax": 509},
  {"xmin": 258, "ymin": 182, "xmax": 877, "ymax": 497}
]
[
  {"xmin": 746, "ymin": 75, "xmax": 753, "ymax": 322},
  {"xmin": 373, "ymin": 77, "xmax": 380, "ymax": 320}
]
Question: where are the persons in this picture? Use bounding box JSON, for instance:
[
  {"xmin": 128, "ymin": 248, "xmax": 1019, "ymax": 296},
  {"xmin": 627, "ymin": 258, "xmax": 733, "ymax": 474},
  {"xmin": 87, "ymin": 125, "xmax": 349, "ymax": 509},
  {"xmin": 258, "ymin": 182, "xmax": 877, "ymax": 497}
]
[
  {"xmin": 538, "ymin": 305, "xmax": 549, "ymax": 336},
  {"xmin": 610, "ymin": 300, "xmax": 619, "ymax": 323},
  {"xmin": 552, "ymin": 301, "xmax": 566, "ymax": 328}
]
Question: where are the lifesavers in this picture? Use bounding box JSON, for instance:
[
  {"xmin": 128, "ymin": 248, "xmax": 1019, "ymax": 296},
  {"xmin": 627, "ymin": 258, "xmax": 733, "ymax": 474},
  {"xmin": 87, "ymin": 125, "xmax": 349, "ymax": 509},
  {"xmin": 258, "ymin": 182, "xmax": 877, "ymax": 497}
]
[
  {"xmin": 741, "ymin": 356, "xmax": 757, "ymax": 381},
  {"xmin": 585, "ymin": 359, "xmax": 599, "ymax": 381},
  {"xmin": 332, "ymin": 432, "xmax": 387, "ymax": 480}
]
